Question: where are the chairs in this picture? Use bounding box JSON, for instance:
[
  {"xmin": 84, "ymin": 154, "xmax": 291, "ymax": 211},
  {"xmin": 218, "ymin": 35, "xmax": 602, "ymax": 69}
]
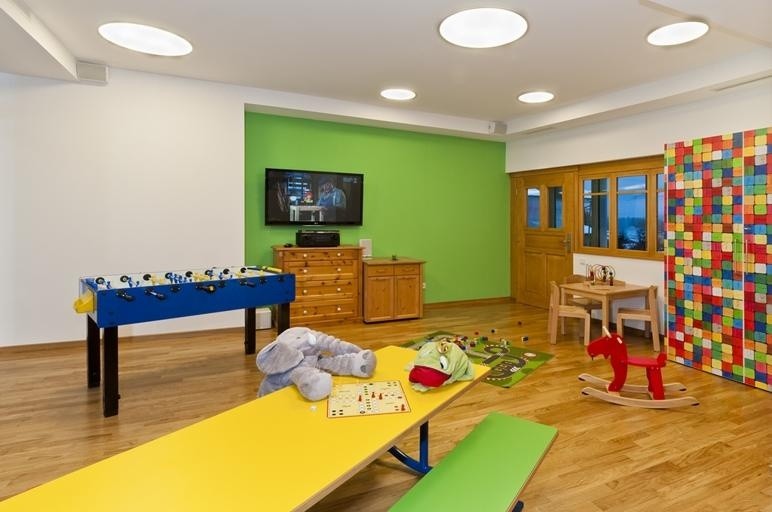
[{"xmin": 543, "ymin": 274, "xmax": 660, "ymax": 353}]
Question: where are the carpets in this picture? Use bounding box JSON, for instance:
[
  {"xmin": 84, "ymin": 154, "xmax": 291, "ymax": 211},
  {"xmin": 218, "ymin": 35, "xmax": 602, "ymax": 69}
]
[{"xmin": 396, "ymin": 328, "xmax": 557, "ymax": 391}]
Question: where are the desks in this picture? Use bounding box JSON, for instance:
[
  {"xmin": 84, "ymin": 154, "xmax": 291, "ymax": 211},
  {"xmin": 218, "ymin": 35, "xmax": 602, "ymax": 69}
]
[{"xmin": 1, "ymin": 343, "xmax": 493, "ymax": 512}]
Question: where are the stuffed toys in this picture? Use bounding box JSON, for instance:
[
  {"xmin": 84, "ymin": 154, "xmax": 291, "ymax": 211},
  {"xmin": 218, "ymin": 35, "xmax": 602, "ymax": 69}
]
[
  {"xmin": 248, "ymin": 322, "xmax": 377, "ymax": 404},
  {"xmin": 405, "ymin": 336, "xmax": 477, "ymax": 396}
]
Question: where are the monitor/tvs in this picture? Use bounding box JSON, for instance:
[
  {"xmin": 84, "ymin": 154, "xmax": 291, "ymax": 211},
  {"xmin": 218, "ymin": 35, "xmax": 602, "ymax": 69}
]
[{"xmin": 264, "ymin": 167, "xmax": 365, "ymax": 223}]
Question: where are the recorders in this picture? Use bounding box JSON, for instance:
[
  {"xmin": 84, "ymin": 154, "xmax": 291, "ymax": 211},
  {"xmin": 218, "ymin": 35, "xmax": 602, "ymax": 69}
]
[{"xmin": 296, "ymin": 229, "xmax": 340, "ymax": 247}]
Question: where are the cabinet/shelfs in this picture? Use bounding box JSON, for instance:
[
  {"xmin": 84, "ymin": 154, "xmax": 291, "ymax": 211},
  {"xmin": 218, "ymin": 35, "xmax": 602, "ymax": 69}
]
[
  {"xmin": 363, "ymin": 255, "xmax": 426, "ymax": 325},
  {"xmin": 270, "ymin": 243, "xmax": 365, "ymax": 329}
]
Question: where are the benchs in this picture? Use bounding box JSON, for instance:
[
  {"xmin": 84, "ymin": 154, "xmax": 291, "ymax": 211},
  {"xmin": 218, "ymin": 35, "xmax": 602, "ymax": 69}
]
[{"xmin": 384, "ymin": 408, "xmax": 562, "ymax": 510}]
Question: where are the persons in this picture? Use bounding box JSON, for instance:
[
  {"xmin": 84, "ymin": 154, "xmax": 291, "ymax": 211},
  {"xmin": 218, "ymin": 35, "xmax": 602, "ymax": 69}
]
[
  {"xmin": 315, "ymin": 179, "xmax": 346, "ymax": 211},
  {"xmin": 304, "ymin": 192, "xmax": 314, "ymax": 205}
]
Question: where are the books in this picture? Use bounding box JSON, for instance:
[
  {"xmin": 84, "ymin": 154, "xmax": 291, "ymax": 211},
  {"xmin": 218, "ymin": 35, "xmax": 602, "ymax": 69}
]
[{"xmin": 326, "ymin": 379, "xmax": 414, "ymax": 422}]
